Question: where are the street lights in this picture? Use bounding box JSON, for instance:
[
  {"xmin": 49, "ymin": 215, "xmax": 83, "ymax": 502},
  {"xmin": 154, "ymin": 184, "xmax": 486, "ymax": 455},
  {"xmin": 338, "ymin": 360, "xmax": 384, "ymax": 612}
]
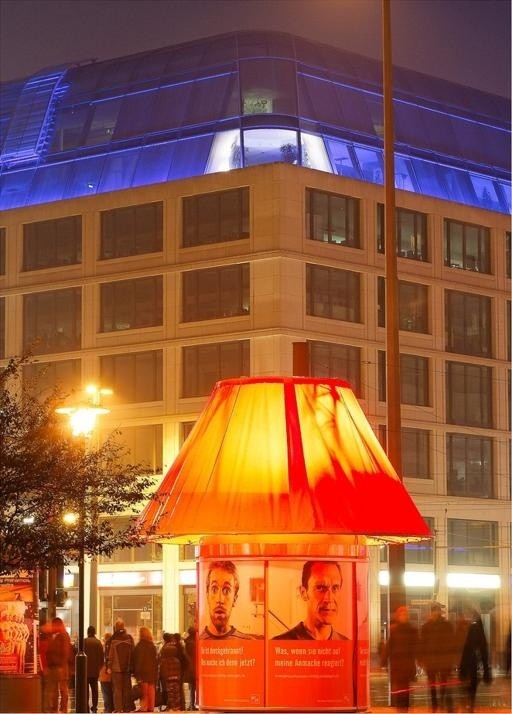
[
  {"xmin": 51, "ymin": 402, "xmax": 110, "ymax": 714},
  {"xmin": 84, "ymin": 383, "xmax": 116, "ymax": 641}
]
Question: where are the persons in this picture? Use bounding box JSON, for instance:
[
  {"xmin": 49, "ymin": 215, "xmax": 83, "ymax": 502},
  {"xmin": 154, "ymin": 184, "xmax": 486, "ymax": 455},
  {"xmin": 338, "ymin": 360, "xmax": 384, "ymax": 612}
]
[
  {"xmin": 269, "ymin": 561, "xmax": 350, "ymax": 639},
  {"xmin": 417, "ymin": 599, "xmax": 457, "ymax": 713},
  {"xmin": 36, "ymin": 618, "xmax": 201, "ymax": 712},
  {"xmin": 381, "ymin": 604, "xmax": 417, "ymax": 713},
  {"xmin": 199, "ymin": 561, "xmax": 260, "ymax": 641},
  {"xmin": 456, "ymin": 599, "xmax": 492, "ymax": 713}
]
